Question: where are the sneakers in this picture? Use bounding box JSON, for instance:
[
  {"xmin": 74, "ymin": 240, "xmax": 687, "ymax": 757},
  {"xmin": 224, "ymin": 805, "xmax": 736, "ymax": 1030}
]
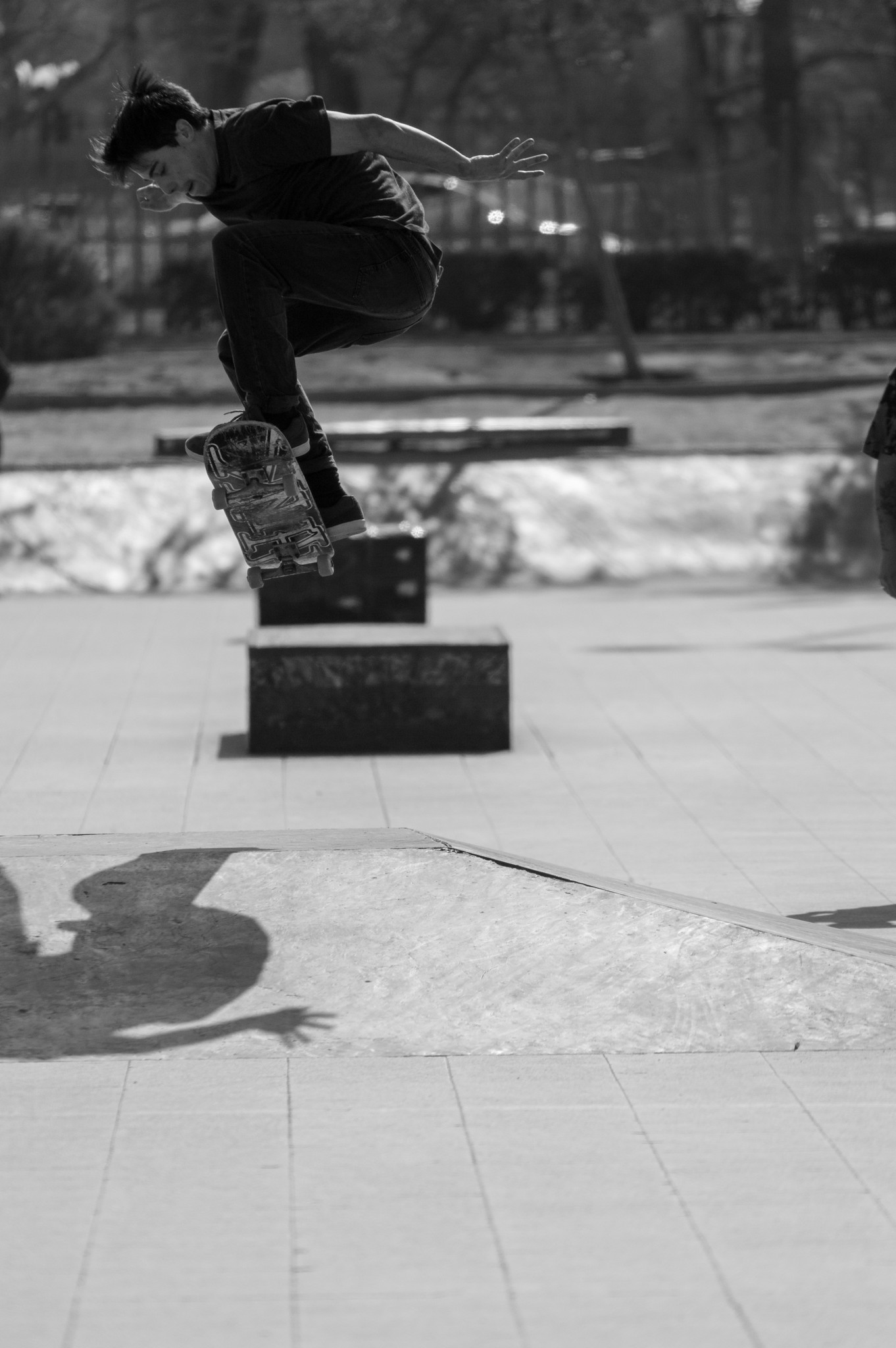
[
  {"xmin": 185, "ymin": 411, "xmax": 310, "ymax": 462},
  {"xmin": 321, "ymin": 494, "xmax": 366, "ymax": 543}
]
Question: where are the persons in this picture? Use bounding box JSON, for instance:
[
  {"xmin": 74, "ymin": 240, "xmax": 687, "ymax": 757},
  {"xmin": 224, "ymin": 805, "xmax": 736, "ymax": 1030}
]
[
  {"xmin": 862, "ymin": 368, "xmax": 896, "ymax": 599},
  {"xmin": 89, "ymin": 66, "xmax": 549, "ymax": 542}
]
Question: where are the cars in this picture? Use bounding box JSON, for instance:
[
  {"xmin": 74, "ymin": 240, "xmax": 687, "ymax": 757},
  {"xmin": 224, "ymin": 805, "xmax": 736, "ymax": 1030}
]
[{"xmin": 154, "ymin": 170, "xmax": 632, "ymax": 333}]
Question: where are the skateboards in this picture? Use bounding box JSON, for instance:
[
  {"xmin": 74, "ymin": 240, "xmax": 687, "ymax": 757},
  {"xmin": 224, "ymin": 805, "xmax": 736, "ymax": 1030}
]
[{"xmin": 204, "ymin": 421, "xmax": 335, "ymax": 590}]
